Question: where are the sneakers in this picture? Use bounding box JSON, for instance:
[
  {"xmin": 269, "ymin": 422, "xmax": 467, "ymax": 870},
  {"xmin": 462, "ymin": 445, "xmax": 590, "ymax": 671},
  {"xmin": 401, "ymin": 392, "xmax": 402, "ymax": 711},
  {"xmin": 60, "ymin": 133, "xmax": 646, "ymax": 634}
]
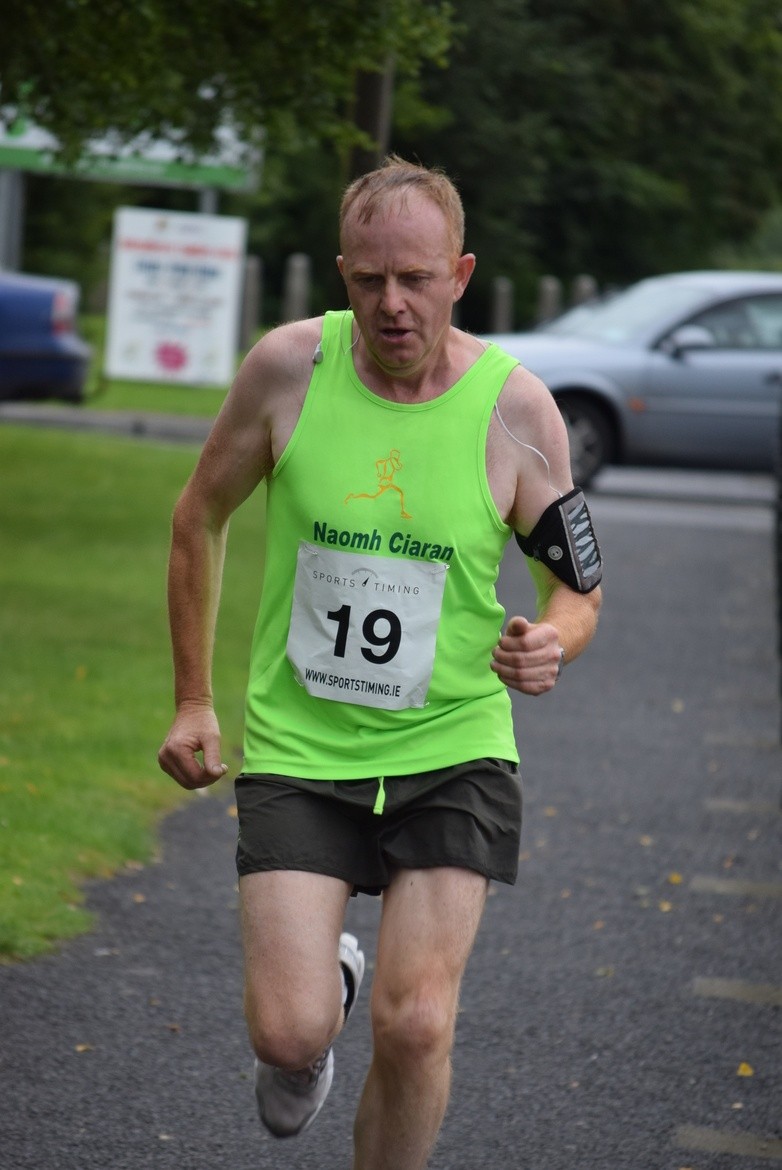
[{"xmin": 255, "ymin": 933, "xmax": 366, "ymax": 1140}]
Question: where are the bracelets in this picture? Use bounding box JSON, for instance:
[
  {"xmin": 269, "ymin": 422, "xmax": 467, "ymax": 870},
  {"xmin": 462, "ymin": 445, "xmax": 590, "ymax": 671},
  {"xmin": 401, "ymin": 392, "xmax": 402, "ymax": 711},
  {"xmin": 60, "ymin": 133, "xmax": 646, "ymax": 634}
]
[{"xmin": 558, "ymin": 643, "xmax": 565, "ymax": 678}]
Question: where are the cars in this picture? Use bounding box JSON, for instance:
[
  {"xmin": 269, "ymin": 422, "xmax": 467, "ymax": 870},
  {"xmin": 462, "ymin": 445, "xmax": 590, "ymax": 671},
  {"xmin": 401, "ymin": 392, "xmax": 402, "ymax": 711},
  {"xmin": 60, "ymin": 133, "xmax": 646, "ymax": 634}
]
[
  {"xmin": 478, "ymin": 270, "xmax": 782, "ymax": 488},
  {"xmin": 0, "ymin": 272, "xmax": 92, "ymax": 401}
]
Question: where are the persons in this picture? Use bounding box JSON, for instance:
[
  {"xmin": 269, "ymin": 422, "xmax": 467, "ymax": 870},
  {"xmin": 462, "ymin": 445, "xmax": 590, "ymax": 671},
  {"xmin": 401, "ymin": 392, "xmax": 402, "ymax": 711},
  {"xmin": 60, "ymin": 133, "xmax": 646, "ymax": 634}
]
[{"xmin": 157, "ymin": 155, "xmax": 602, "ymax": 1170}]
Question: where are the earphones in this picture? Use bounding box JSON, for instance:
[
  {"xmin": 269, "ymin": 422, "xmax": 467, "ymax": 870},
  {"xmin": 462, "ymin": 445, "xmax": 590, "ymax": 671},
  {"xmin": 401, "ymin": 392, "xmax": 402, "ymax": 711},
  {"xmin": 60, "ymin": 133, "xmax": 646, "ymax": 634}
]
[{"xmin": 312, "ymin": 343, "xmax": 324, "ymax": 364}]
